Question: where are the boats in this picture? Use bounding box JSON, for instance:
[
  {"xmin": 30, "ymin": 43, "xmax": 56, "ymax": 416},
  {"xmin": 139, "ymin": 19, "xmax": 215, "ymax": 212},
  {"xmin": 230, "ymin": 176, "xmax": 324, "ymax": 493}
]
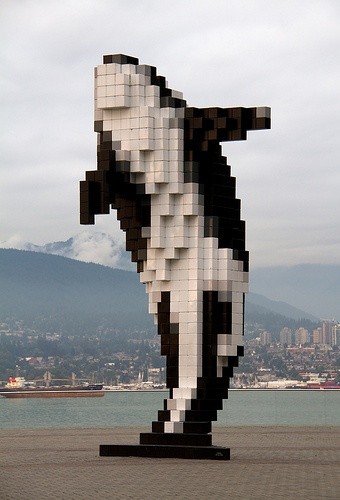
[{"xmin": 0, "ymin": 364, "xmax": 105, "ymax": 398}]
